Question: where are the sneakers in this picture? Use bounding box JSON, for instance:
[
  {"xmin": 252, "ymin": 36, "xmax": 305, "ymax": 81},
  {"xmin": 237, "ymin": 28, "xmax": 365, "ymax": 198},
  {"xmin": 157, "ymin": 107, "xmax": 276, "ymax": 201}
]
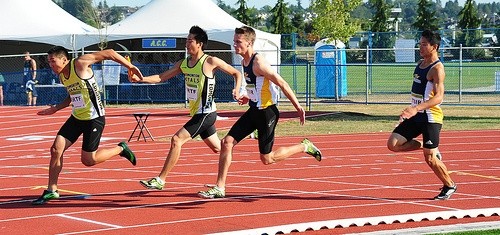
[
  {"xmin": 139, "ymin": 178, "xmax": 164, "ymax": 190},
  {"xmin": 435, "ymin": 147, "xmax": 441, "ymax": 161},
  {"xmin": 197, "ymin": 187, "xmax": 226, "ymax": 198},
  {"xmin": 434, "ymin": 181, "xmax": 457, "ymax": 201},
  {"xmin": 253, "ymin": 129, "xmax": 259, "ymax": 139},
  {"xmin": 118, "ymin": 141, "xmax": 137, "ymax": 166},
  {"xmin": 31, "ymin": 189, "xmax": 59, "ymax": 205},
  {"xmin": 300, "ymin": 138, "xmax": 321, "ymax": 162}
]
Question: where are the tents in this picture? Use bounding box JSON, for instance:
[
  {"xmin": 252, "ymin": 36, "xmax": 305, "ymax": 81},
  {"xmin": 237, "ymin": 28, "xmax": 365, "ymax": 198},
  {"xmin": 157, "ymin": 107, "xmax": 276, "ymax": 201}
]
[{"xmin": 0, "ymin": 0, "xmax": 283, "ymax": 102}]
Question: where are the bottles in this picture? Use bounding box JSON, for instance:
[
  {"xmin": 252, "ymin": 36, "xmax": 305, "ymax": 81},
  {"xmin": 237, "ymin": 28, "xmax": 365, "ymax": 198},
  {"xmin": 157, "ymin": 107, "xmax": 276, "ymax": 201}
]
[{"xmin": 52, "ymin": 78, "xmax": 55, "ymax": 87}]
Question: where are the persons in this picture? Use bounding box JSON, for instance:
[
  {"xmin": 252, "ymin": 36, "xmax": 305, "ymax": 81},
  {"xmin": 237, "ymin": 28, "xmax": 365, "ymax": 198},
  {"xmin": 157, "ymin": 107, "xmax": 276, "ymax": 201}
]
[
  {"xmin": 387, "ymin": 31, "xmax": 458, "ymax": 201},
  {"xmin": 130, "ymin": 26, "xmax": 242, "ymax": 190},
  {"xmin": 197, "ymin": 27, "xmax": 322, "ymax": 199},
  {"xmin": 30, "ymin": 46, "xmax": 143, "ymax": 206},
  {"xmin": 0, "ymin": 50, "xmax": 185, "ymax": 107}
]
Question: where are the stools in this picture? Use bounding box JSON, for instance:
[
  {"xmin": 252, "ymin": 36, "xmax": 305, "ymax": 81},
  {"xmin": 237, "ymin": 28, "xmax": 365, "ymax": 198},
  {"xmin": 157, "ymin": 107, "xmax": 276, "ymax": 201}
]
[{"xmin": 127, "ymin": 112, "xmax": 154, "ymax": 143}]
